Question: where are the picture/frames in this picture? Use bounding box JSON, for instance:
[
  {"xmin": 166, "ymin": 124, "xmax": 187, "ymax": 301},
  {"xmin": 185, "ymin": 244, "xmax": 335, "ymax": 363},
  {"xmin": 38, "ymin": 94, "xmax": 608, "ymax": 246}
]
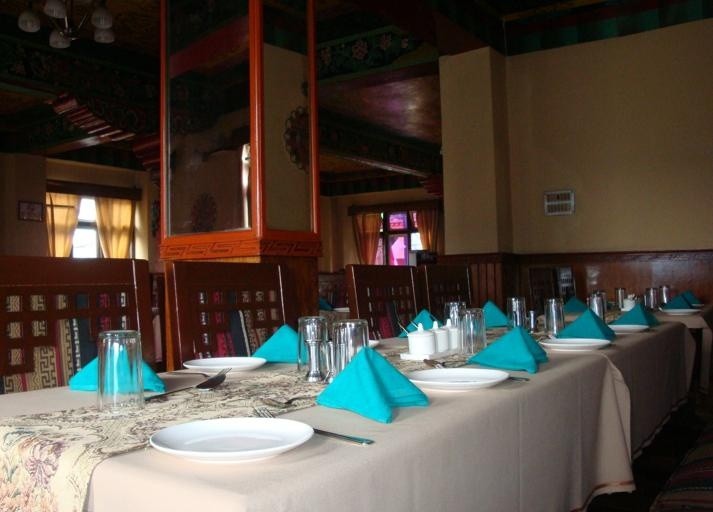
[{"xmin": 18, "ymin": 200, "xmax": 44, "ymax": 223}]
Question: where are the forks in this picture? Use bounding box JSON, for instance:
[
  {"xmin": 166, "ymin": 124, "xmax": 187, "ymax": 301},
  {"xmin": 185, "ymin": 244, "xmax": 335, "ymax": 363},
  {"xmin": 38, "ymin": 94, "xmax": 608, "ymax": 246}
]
[
  {"xmin": 423, "ymin": 358, "xmax": 530, "ymax": 384},
  {"xmin": 251, "ymin": 407, "xmax": 375, "ymax": 447},
  {"xmin": 168, "ymin": 367, "xmax": 231, "ymax": 379},
  {"xmin": 262, "ymin": 396, "xmax": 319, "ymax": 407}
]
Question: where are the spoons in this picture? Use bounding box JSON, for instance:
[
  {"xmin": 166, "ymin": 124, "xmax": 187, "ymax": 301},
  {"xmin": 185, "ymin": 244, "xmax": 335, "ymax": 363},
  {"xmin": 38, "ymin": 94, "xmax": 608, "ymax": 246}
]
[{"xmin": 143, "ymin": 374, "xmax": 226, "ymax": 402}]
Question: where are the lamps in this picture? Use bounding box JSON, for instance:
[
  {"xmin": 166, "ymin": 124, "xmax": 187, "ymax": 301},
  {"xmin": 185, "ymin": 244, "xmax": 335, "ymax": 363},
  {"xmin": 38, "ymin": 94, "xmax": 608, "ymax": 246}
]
[{"xmin": 17, "ymin": 0, "xmax": 115, "ymax": 49}]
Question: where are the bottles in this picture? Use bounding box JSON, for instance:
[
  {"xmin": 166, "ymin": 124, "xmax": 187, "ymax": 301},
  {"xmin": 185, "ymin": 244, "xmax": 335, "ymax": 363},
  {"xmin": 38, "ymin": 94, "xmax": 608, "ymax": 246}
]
[
  {"xmin": 441, "ymin": 318, "xmax": 458, "ymax": 349},
  {"xmin": 407, "ymin": 322, "xmax": 435, "ymax": 354},
  {"xmin": 427, "ymin": 321, "xmax": 449, "ymax": 353},
  {"xmin": 528, "ymin": 310, "xmax": 538, "ymax": 334}
]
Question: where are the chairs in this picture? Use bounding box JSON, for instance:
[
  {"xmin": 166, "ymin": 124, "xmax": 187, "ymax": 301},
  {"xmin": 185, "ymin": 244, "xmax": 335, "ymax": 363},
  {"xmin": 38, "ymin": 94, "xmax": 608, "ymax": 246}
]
[
  {"xmin": 161, "ymin": 260, "xmax": 297, "ymax": 370},
  {"xmin": 344, "ymin": 263, "xmax": 416, "ymax": 337},
  {"xmin": 417, "ymin": 263, "xmax": 473, "ymax": 319},
  {"xmin": 0, "ymin": 254, "xmax": 156, "ymax": 395},
  {"xmin": 553, "ymin": 264, "xmax": 578, "ymax": 305},
  {"xmin": 525, "ymin": 266, "xmax": 556, "ymax": 311}
]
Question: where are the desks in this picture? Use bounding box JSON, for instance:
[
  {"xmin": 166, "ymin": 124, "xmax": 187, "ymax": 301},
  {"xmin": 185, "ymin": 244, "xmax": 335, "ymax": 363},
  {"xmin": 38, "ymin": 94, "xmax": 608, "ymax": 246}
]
[{"xmin": 0, "ymin": 302, "xmax": 712, "ymax": 510}]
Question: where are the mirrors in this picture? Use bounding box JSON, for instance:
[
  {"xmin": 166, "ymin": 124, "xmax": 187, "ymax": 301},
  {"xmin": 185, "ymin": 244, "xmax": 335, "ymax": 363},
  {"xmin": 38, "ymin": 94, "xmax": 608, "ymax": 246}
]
[
  {"xmin": 159, "ymin": 0, "xmax": 260, "ymax": 244},
  {"xmin": 252, "ymin": 0, "xmax": 319, "ymax": 242}
]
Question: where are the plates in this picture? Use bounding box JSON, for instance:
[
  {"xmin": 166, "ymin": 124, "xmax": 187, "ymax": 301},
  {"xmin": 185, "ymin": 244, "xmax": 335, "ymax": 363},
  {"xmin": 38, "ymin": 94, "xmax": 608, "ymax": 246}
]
[
  {"xmin": 148, "ymin": 416, "xmax": 314, "ymax": 462},
  {"xmin": 539, "ymin": 338, "xmax": 611, "ymax": 351},
  {"xmin": 662, "ymin": 309, "xmax": 702, "ymax": 315},
  {"xmin": 690, "ymin": 303, "xmax": 704, "ymax": 306},
  {"xmin": 329, "ymin": 340, "xmax": 379, "ymax": 351},
  {"xmin": 407, "ymin": 368, "xmax": 510, "ymax": 393},
  {"xmin": 184, "ymin": 355, "xmax": 266, "ymax": 373},
  {"xmin": 608, "ymin": 324, "xmax": 650, "ymax": 335}
]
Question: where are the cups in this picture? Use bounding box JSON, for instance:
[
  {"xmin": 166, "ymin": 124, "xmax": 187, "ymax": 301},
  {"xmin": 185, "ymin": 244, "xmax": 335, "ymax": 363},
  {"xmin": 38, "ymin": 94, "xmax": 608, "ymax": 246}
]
[
  {"xmin": 443, "ymin": 302, "xmax": 467, "ymax": 352},
  {"xmin": 544, "ymin": 298, "xmax": 565, "ymax": 334},
  {"xmin": 614, "ymin": 288, "xmax": 625, "ymax": 308},
  {"xmin": 586, "ymin": 294, "xmax": 604, "ymax": 322},
  {"xmin": 297, "ymin": 314, "xmax": 332, "ymax": 383},
  {"xmin": 463, "ymin": 307, "xmax": 488, "ymax": 354},
  {"xmin": 506, "ymin": 296, "xmax": 526, "ymax": 331},
  {"xmin": 659, "ymin": 285, "xmax": 669, "ymax": 305},
  {"xmin": 644, "ymin": 287, "xmax": 657, "ymax": 311},
  {"xmin": 333, "ymin": 319, "xmax": 369, "ymax": 378},
  {"xmin": 97, "ymin": 330, "xmax": 145, "ymax": 414},
  {"xmin": 592, "ymin": 290, "xmax": 607, "ymax": 313},
  {"xmin": 621, "ymin": 300, "xmax": 636, "ymax": 311}
]
